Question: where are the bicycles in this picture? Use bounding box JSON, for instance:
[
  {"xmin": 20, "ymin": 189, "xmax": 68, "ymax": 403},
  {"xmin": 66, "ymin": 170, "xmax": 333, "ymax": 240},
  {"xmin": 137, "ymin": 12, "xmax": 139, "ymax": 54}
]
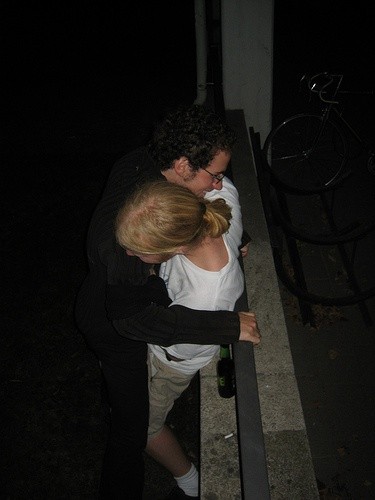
[{"xmin": 261, "ymin": 70, "xmax": 375, "ymax": 195}]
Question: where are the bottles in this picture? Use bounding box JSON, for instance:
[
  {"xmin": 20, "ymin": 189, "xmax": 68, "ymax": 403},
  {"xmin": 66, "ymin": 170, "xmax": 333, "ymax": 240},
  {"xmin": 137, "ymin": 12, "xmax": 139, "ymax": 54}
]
[{"xmin": 217, "ymin": 344, "xmax": 237, "ymax": 398}]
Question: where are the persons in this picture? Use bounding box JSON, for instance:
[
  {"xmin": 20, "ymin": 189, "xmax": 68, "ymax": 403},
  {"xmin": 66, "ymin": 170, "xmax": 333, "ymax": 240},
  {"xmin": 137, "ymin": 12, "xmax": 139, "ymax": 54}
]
[
  {"xmin": 112, "ymin": 174, "xmax": 246, "ymax": 500},
  {"xmin": 74, "ymin": 105, "xmax": 262, "ymax": 500}
]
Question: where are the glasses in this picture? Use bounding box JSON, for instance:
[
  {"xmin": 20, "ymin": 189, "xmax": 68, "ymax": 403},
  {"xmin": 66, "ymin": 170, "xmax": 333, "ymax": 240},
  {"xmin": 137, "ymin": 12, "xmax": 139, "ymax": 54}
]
[{"xmin": 201, "ymin": 166, "xmax": 225, "ymax": 186}]
